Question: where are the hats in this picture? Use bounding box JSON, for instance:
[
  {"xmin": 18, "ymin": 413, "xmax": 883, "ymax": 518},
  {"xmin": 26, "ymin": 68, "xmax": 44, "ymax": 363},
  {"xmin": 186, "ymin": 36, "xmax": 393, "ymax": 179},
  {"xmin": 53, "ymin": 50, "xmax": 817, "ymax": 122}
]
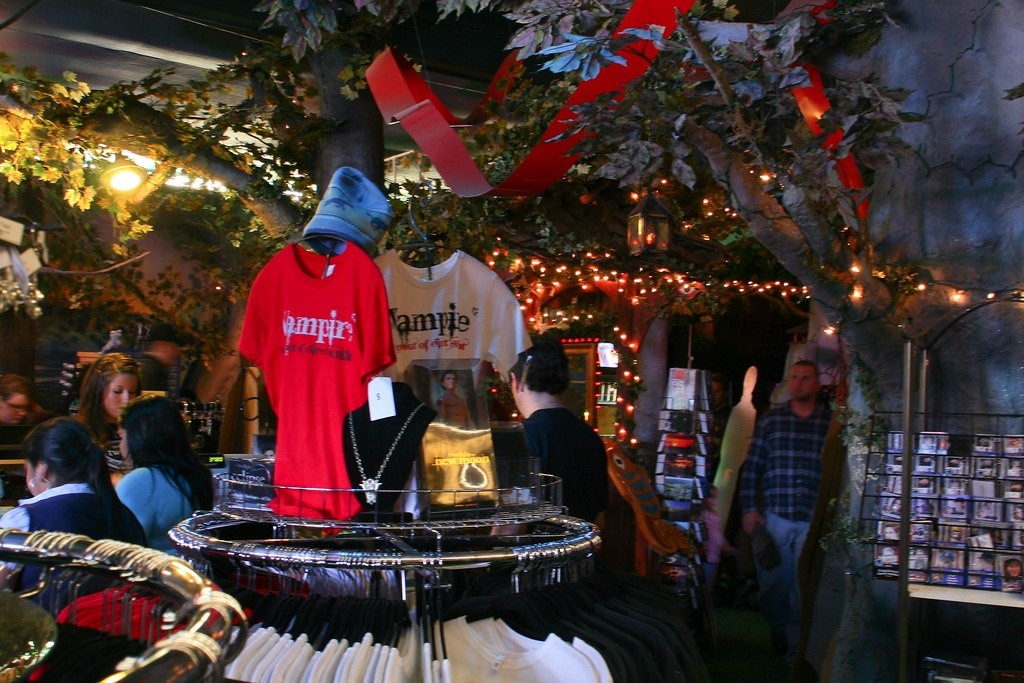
[
  {"xmin": 302, "ymin": 167, "xmax": 394, "ymax": 258},
  {"xmin": 146, "ymin": 322, "xmax": 180, "ymax": 343}
]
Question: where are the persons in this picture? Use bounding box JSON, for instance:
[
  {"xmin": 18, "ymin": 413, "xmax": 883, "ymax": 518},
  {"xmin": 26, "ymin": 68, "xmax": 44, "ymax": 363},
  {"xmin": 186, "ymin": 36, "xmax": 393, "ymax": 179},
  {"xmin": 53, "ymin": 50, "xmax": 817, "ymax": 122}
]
[
  {"xmin": 489, "ymin": 338, "xmax": 609, "ymax": 558},
  {"xmin": 0, "ymin": 373, "xmax": 43, "ymax": 426},
  {"xmin": 977, "ymin": 553, "xmax": 996, "ymax": 571},
  {"xmin": 736, "ymin": 360, "xmax": 834, "ymax": 683},
  {"xmin": 873, "ymin": 499, "xmax": 901, "ymax": 567},
  {"xmin": 113, "ymin": 393, "xmax": 214, "ymax": 559},
  {"xmin": 890, "ymin": 455, "xmax": 961, "ymax": 492},
  {"xmin": 976, "ymin": 437, "xmax": 1024, "ymax": 545},
  {"xmin": 1003, "ymin": 558, "xmax": 1023, "ymax": 584},
  {"xmin": 139, "ymin": 341, "xmax": 200, "ymax": 447},
  {"xmin": 0, "ymin": 417, "xmax": 147, "ymax": 617},
  {"xmin": 912, "ymin": 498, "xmax": 965, "ymax": 583},
  {"xmin": 74, "ymin": 352, "xmax": 141, "ymax": 489}
]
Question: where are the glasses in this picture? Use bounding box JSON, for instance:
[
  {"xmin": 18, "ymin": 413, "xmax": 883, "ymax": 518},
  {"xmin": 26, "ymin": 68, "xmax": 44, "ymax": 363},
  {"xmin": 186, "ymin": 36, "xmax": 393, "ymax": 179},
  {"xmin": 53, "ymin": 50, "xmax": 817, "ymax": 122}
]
[
  {"xmin": 521, "ymin": 348, "xmax": 536, "ymax": 392},
  {"xmin": 2, "ymin": 398, "xmax": 30, "ymax": 414}
]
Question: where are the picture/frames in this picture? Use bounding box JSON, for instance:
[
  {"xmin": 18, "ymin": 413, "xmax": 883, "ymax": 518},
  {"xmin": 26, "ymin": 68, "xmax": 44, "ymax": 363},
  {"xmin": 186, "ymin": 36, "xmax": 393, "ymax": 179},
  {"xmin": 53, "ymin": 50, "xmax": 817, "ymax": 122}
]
[{"xmin": 567, "ymin": 343, "xmax": 597, "ymax": 429}]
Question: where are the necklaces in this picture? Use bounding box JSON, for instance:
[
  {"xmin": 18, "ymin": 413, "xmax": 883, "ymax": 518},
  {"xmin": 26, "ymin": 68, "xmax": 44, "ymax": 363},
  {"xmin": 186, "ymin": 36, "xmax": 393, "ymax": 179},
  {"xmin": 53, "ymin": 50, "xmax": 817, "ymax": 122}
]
[{"xmin": 349, "ymin": 403, "xmax": 425, "ymax": 504}]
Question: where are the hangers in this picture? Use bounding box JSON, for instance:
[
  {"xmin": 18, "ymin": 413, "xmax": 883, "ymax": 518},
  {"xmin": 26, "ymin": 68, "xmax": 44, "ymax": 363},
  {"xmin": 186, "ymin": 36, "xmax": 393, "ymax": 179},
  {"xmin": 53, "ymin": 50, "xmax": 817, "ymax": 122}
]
[{"xmin": 1, "ymin": 471, "xmax": 602, "ymax": 682}]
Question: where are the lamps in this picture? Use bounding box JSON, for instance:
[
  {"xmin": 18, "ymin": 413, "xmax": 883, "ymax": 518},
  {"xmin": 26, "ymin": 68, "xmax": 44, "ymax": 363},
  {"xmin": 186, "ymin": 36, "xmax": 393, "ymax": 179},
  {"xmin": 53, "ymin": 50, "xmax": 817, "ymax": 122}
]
[{"xmin": 101, "ymin": 152, "xmax": 149, "ymax": 195}]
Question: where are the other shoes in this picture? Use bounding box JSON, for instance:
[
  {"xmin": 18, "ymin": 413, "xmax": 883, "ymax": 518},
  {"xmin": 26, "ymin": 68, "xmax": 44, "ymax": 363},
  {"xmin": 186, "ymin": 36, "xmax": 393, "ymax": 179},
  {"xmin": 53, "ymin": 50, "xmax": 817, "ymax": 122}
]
[{"xmin": 771, "ymin": 625, "xmax": 789, "ymax": 655}]
